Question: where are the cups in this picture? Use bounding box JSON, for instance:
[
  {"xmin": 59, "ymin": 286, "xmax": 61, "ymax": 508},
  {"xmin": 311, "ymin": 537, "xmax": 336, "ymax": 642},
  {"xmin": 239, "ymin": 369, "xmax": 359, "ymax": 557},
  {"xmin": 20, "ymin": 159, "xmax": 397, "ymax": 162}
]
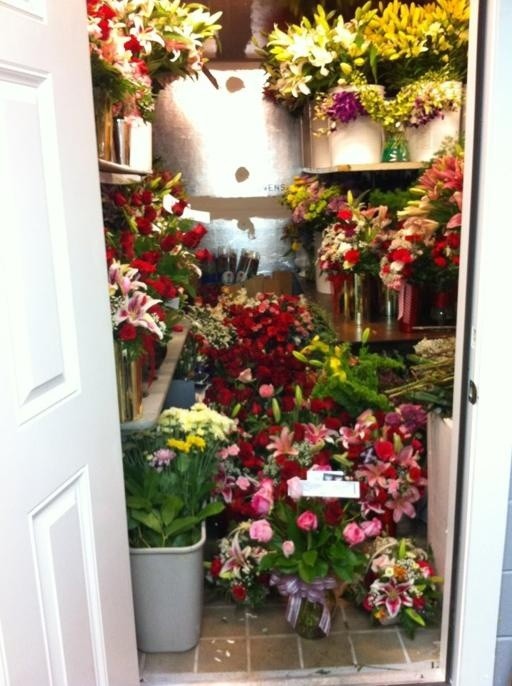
[
  {"xmin": 235, "ymin": 249, "xmax": 260, "ymax": 283},
  {"xmin": 216, "ymin": 246, "xmax": 237, "ymax": 285}
]
